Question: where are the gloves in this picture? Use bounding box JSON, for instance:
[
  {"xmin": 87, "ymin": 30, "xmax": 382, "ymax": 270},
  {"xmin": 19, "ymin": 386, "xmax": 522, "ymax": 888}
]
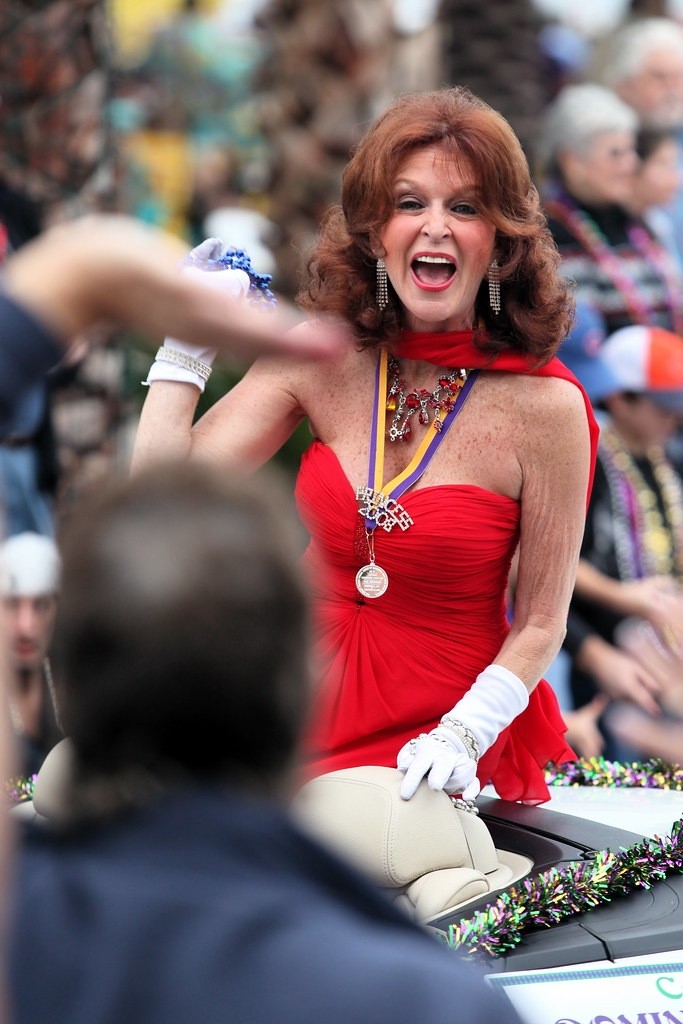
[
  {"xmin": 397, "ymin": 665, "xmax": 529, "ymax": 801},
  {"xmin": 141, "ymin": 237, "xmax": 249, "ymax": 393}
]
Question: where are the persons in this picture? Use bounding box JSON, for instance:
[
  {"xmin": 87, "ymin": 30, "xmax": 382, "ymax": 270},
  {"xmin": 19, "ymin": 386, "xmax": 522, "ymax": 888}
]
[
  {"xmin": 0, "ymin": 451, "xmax": 521, "ymax": 1023},
  {"xmin": 1, "ymin": 1, "xmax": 681, "ymax": 806},
  {"xmin": 132, "ymin": 86, "xmax": 593, "ymax": 802}
]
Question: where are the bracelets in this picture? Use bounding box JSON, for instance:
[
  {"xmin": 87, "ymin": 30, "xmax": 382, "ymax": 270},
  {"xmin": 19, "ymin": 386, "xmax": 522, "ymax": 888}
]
[
  {"xmin": 409, "ymin": 718, "xmax": 480, "ymax": 767},
  {"xmin": 155, "ymin": 345, "xmax": 211, "ymax": 381}
]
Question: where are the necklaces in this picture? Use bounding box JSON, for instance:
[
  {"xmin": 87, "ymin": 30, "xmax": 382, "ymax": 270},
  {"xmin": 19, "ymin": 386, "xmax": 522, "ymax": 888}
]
[
  {"xmin": 541, "ymin": 200, "xmax": 683, "ymax": 330},
  {"xmin": 591, "ymin": 410, "xmax": 682, "ymax": 582},
  {"xmin": 0, "ymin": 660, "xmax": 61, "ymax": 737},
  {"xmin": 376, "ymin": 353, "xmax": 469, "ymax": 444}
]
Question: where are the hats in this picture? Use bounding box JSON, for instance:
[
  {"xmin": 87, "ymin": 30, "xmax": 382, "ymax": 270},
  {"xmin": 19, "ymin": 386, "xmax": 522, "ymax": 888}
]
[
  {"xmin": 600, "ymin": 325, "xmax": 683, "ymax": 414},
  {"xmin": 0, "ymin": 531, "xmax": 63, "ymax": 596},
  {"xmin": 553, "ymin": 305, "xmax": 620, "ymax": 401}
]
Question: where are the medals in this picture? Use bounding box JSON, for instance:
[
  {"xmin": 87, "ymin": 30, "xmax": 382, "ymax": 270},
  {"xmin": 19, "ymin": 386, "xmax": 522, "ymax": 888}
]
[{"xmin": 353, "ymin": 487, "xmax": 412, "ymax": 600}]
